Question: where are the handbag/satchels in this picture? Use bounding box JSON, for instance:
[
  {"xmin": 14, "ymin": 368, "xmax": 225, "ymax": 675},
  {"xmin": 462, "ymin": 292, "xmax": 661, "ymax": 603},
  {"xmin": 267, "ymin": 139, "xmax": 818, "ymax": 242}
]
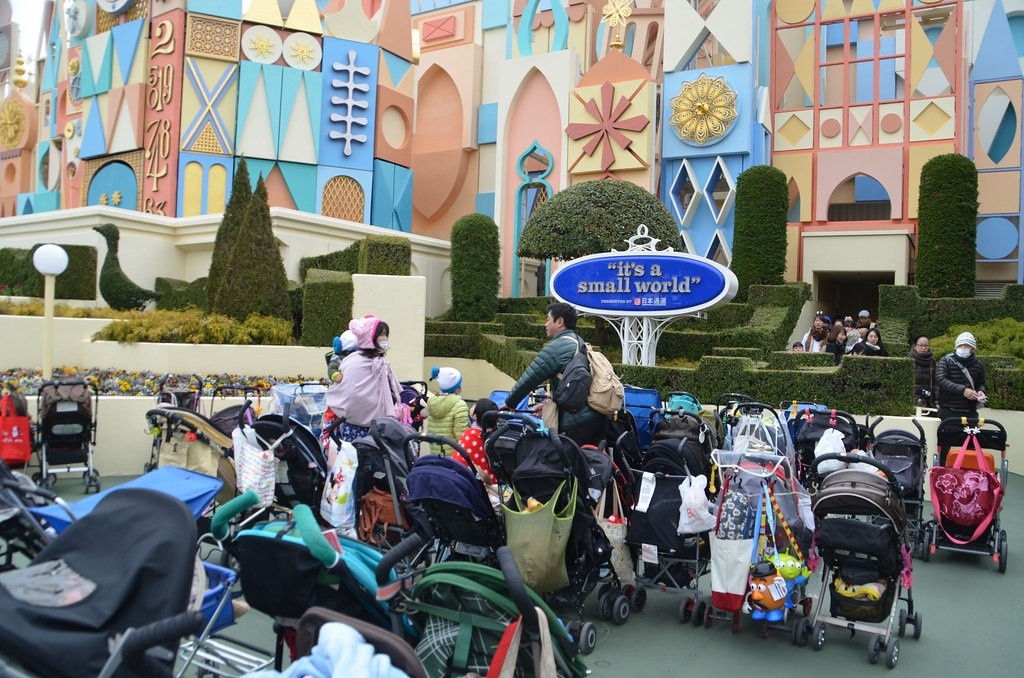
[
  {"xmin": 235, "ymin": 434, "xmax": 276, "ymax": 508},
  {"xmin": 499, "ymin": 474, "xmax": 579, "ymax": 593},
  {"xmin": 708, "ymin": 477, "xmax": 762, "ymax": 611},
  {"xmin": 0, "ymin": 393, "xmax": 30, "ymax": 464},
  {"xmin": 677, "ymin": 475, "xmax": 717, "ymax": 534},
  {"xmin": 874, "ymin": 449, "xmax": 917, "ymax": 489},
  {"xmin": 318, "ymin": 442, "xmax": 358, "ymax": 542},
  {"xmin": 596, "ymin": 480, "xmax": 636, "ymax": 584},
  {"xmin": 552, "ymin": 351, "xmax": 592, "ymax": 415},
  {"xmin": 929, "ymin": 434, "xmax": 1002, "ymax": 545},
  {"xmin": 937, "ymin": 403, "xmax": 978, "ymax": 422},
  {"xmin": 813, "ymin": 429, "xmax": 846, "ymax": 473},
  {"xmin": 732, "ymin": 419, "xmax": 777, "ymax": 456}
]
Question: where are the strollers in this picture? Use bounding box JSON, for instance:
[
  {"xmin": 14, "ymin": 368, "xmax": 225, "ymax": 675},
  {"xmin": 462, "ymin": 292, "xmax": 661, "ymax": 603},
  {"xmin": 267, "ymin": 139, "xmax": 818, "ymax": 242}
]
[
  {"xmin": 30, "ymin": 378, "xmax": 101, "ymax": 488},
  {"xmin": 146, "ymin": 380, "xmax": 1008, "ymax": 668},
  {"xmin": 1, "ymin": 394, "xmax": 592, "ymax": 678}
]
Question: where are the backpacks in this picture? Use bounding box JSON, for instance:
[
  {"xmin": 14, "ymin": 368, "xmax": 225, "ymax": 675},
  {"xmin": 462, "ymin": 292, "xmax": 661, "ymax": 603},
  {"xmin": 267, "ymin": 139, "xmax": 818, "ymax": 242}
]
[
  {"xmin": 394, "ymin": 402, "xmax": 413, "ymax": 427},
  {"xmin": 359, "ymin": 488, "xmax": 408, "ymax": 544},
  {"xmin": 562, "ymin": 336, "xmax": 626, "ymax": 421}
]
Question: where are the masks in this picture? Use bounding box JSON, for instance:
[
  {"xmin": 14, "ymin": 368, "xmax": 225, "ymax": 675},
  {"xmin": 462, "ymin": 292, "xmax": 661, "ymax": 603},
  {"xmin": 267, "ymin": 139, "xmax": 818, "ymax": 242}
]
[
  {"xmin": 376, "ymin": 342, "xmax": 391, "ymax": 354},
  {"xmin": 956, "ymin": 348, "xmax": 973, "ymax": 359}
]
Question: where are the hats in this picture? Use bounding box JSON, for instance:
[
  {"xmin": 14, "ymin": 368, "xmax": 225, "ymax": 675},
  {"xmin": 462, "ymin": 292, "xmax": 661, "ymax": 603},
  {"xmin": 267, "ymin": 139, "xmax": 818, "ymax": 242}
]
[
  {"xmin": 955, "ymin": 332, "xmax": 977, "ymax": 351},
  {"xmin": 859, "ymin": 310, "xmax": 870, "ymax": 317},
  {"xmin": 350, "ymin": 314, "xmax": 383, "ymax": 349},
  {"xmin": 429, "ymin": 365, "xmax": 462, "ymax": 393}
]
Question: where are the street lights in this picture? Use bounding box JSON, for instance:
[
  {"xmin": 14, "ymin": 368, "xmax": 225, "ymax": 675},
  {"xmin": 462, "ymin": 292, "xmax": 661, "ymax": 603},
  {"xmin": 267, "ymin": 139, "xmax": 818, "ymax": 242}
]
[{"xmin": 33, "ymin": 240, "xmax": 69, "ymax": 381}]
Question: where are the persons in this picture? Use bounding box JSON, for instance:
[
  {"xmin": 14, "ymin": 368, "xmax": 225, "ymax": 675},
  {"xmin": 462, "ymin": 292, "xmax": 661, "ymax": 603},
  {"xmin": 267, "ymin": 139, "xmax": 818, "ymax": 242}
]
[
  {"xmin": 0, "ymin": 382, "xmax": 32, "ymax": 473},
  {"xmin": 451, "ymin": 398, "xmax": 503, "ymax": 487},
  {"xmin": 426, "ymin": 367, "xmax": 470, "ymax": 458},
  {"xmin": 907, "ymin": 335, "xmax": 940, "ymax": 418},
  {"xmin": 793, "ymin": 309, "xmax": 888, "ymax": 357},
  {"xmin": 320, "ymin": 314, "xmax": 404, "ymax": 461},
  {"xmin": 498, "ymin": 303, "xmax": 625, "ymax": 446},
  {"xmin": 934, "ymin": 332, "xmax": 988, "ymax": 421}
]
